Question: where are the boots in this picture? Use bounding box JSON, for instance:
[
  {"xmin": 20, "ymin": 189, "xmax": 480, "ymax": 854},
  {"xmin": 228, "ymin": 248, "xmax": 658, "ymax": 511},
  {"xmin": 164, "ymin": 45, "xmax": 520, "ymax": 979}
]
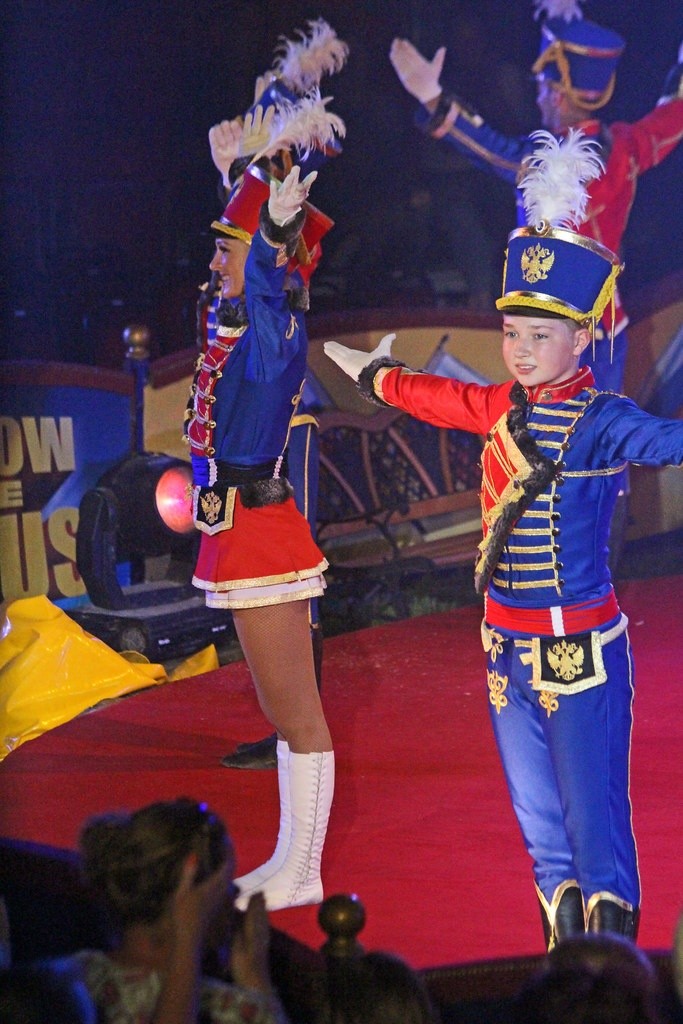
[
  {"xmin": 609, "ymin": 488, "xmax": 631, "ymax": 597},
  {"xmin": 233, "ymin": 740, "xmax": 336, "ymax": 913},
  {"xmin": 220, "ymin": 622, "xmax": 323, "ymax": 770},
  {"xmin": 529, "ymin": 877, "xmax": 640, "ymax": 956}
]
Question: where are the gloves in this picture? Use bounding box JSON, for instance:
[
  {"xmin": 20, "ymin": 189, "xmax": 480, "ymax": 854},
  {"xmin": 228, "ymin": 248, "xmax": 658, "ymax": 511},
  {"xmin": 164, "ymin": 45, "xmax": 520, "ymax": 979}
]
[
  {"xmin": 322, "ymin": 332, "xmax": 398, "ymax": 383},
  {"xmin": 386, "ymin": 37, "xmax": 447, "ymax": 105},
  {"xmin": 238, "ymin": 105, "xmax": 276, "ymax": 189},
  {"xmin": 267, "ymin": 165, "xmax": 319, "ymax": 229},
  {"xmin": 209, "ymin": 119, "xmax": 243, "ymax": 189}
]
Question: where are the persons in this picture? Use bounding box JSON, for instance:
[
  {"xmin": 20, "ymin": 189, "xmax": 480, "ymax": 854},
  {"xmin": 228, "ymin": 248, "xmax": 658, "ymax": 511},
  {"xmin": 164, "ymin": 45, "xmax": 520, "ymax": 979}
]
[
  {"xmin": 2, "ymin": 793, "xmax": 680, "ymax": 1024},
  {"xmin": 0, "ymin": 0, "xmax": 680, "ymax": 350},
  {"xmin": 386, "ymin": 13, "xmax": 680, "ymax": 393},
  {"xmin": 317, "ymin": 221, "xmax": 683, "ymax": 951},
  {"xmin": 173, "ymin": 160, "xmax": 339, "ymax": 915}
]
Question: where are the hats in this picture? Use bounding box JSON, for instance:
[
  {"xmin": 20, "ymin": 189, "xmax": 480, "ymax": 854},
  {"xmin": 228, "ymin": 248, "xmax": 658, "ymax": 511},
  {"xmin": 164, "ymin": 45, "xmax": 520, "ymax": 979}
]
[
  {"xmin": 494, "ymin": 125, "xmax": 625, "ymax": 364},
  {"xmin": 248, "ymin": 20, "xmax": 344, "ymax": 171},
  {"xmin": 211, "ymin": 85, "xmax": 348, "ymax": 277},
  {"xmin": 528, "ymin": 0, "xmax": 624, "ymax": 111},
  {"xmin": 288, "ymin": 240, "xmax": 322, "ymax": 297}
]
